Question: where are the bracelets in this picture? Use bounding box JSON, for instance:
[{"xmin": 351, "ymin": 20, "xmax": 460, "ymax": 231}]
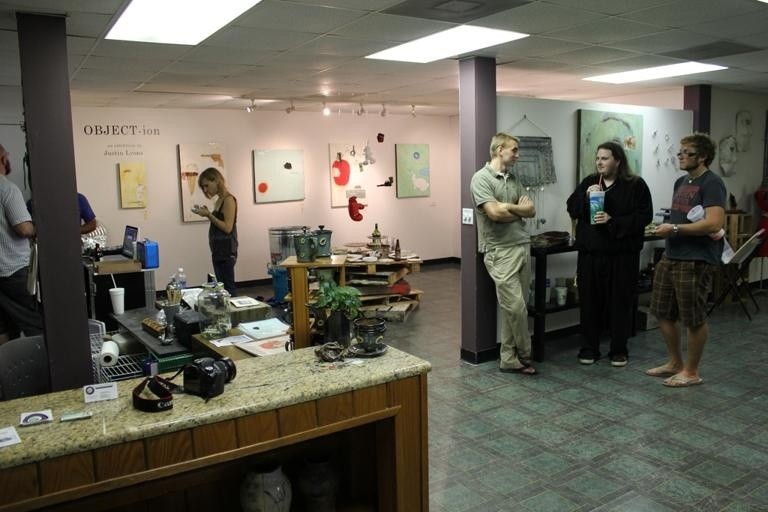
[
  {"xmin": 208, "ymin": 213, "xmax": 217, "ymax": 225},
  {"xmin": 673, "ymin": 223, "xmax": 679, "ymax": 233}
]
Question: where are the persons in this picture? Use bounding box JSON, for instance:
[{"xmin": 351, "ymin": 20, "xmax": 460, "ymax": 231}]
[
  {"xmin": 26, "ymin": 193, "xmax": 96, "ymax": 299},
  {"xmin": 0, "ymin": 146, "xmax": 44, "ymax": 341},
  {"xmin": 470, "ymin": 134, "xmax": 536, "ymax": 375},
  {"xmin": 192, "ymin": 168, "xmax": 238, "ymax": 297},
  {"xmin": 645, "ymin": 134, "xmax": 727, "ymax": 387},
  {"xmin": 566, "ymin": 142, "xmax": 652, "ymax": 367}
]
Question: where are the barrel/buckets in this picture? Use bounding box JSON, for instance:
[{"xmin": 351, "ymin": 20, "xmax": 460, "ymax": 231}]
[{"xmin": 268, "ymin": 225, "xmax": 312, "ymax": 269}]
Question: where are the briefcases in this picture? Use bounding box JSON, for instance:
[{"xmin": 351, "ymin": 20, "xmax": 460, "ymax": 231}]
[{"xmin": 136, "ymin": 237, "xmax": 160, "ymax": 269}]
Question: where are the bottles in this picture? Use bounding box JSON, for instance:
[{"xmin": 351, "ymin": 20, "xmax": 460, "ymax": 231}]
[
  {"xmin": 92, "ymin": 244, "xmax": 103, "ymax": 261},
  {"xmin": 131, "ymin": 241, "xmax": 137, "ymax": 261},
  {"xmin": 373, "ymin": 223, "xmax": 381, "ymax": 245},
  {"xmin": 198, "ymin": 282, "xmax": 232, "ymax": 340},
  {"xmin": 394, "ymin": 239, "xmax": 402, "ymax": 261},
  {"xmin": 240, "ymin": 458, "xmax": 340, "ymax": 510},
  {"xmin": 165, "ymin": 268, "xmax": 186, "ymax": 303}
]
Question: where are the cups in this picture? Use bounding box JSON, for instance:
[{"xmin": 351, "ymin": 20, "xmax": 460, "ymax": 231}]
[
  {"xmin": 543, "ymin": 277, "xmax": 571, "ymax": 308},
  {"xmin": 686, "ymin": 205, "xmax": 726, "ymax": 242},
  {"xmin": 108, "ymin": 287, "xmax": 125, "ymax": 315},
  {"xmin": 292, "ymin": 226, "xmax": 335, "ymax": 262},
  {"xmin": 163, "ymin": 303, "xmax": 180, "ymax": 339},
  {"xmin": 590, "ymin": 192, "xmax": 606, "ymax": 225}
]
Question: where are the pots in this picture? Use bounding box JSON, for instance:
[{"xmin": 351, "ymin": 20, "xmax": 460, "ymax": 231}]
[{"xmin": 268, "ymin": 227, "xmax": 311, "ymax": 265}]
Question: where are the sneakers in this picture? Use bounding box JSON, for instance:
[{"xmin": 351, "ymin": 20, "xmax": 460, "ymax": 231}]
[
  {"xmin": 577, "ymin": 345, "xmax": 600, "ymax": 364},
  {"xmin": 610, "ymin": 347, "xmax": 627, "ymax": 366}
]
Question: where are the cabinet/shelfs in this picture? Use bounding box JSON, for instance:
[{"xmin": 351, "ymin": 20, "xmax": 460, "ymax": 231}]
[
  {"xmin": 525, "ymin": 234, "xmax": 666, "ymax": 364},
  {"xmin": 346, "ymin": 251, "xmax": 423, "ymax": 322},
  {"xmin": 278, "ymin": 255, "xmax": 346, "ymax": 351}
]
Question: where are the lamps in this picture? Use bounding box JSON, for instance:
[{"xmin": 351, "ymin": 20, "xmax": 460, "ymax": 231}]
[{"xmin": 233, "ymin": 95, "xmax": 431, "ymax": 120}]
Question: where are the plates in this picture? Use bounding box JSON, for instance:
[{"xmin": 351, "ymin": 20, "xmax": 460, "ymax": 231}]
[
  {"xmin": 347, "ymin": 344, "xmax": 389, "ymax": 356},
  {"xmin": 345, "ymin": 254, "xmax": 382, "ymax": 263},
  {"xmin": 388, "ymin": 253, "xmax": 412, "ymax": 259}
]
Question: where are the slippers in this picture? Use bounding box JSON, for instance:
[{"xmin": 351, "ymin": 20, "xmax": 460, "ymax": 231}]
[
  {"xmin": 500, "ymin": 357, "xmax": 537, "ymax": 375},
  {"xmin": 661, "ymin": 374, "xmax": 704, "ymax": 388},
  {"xmin": 645, "ymin": 365, "xmax": 680, "ymax": 376}
]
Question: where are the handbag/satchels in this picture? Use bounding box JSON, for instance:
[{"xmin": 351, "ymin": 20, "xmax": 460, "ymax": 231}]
[{"xmin": 212, "ymin": 239, "xmax": 231, "ymax": 266}]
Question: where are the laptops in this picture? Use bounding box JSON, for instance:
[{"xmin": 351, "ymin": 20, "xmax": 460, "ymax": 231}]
[{"xmin": 100, "ymin": 225, "xmax": 140, "ymax": 263}]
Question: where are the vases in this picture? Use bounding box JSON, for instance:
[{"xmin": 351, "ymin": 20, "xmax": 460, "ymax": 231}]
[
  {"xmin": 299, "ymin": 461, "xmax": 339, "ymax": 512},
  {"xmin": 240, "ymin": 461, "xmax": 292, "ymax": 511}
]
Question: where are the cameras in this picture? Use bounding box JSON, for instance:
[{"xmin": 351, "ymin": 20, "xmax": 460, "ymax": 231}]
[{"xmin": 183, "ymin": 356, "xmax": 237, "ymax": 402}]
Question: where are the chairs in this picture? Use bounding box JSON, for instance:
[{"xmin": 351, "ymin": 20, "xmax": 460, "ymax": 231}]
[
  {"xmin": 709, "ymin": 227, "xmax": 766, "ymax": 321},
  {"xmin": 0, "ymin": 333, "xmax": 51, "ymax": 402}
]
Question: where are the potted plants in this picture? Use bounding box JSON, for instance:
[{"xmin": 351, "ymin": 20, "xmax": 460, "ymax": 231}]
[{"xmin": 305, "ymin": 273, "xmax": 362, "ymax": 348}]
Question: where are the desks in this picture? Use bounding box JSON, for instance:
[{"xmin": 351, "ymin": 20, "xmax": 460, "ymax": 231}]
[
  {"xmin": 0, "ymin": 341, "xmax": 432, "ymax": 512},
  {"xmin": 191, "ymin": 325, "xmax": 258, "ymax": 362},
  {"xmin": 84, "ymin": 260, "xmax": 158, "ymax": 319}
]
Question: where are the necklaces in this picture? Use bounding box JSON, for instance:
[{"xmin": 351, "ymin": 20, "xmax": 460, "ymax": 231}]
[{"xmin": 605, "ymin": 184, "xmax": 616, "ymax": 193}]
